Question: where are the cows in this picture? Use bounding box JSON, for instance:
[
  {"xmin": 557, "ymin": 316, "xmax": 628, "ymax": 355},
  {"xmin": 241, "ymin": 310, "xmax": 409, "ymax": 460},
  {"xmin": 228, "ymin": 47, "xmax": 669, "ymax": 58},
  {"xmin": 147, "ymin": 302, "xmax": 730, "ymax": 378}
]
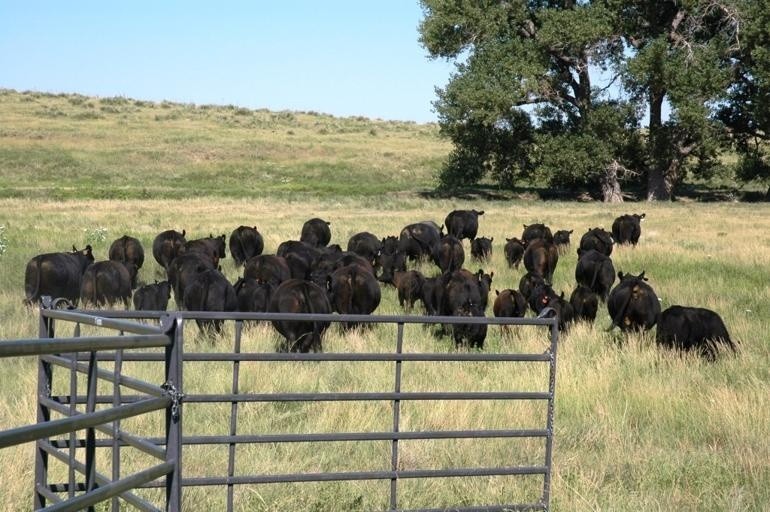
[
  {"xmin": 612, "ymin": 214, "xmax": 646, "ymax": 247},
  {"xmin": 493, "ymin": 289, "xmax": 527, "ymax": 340},
  {"xmin": 445, "ymin": 209, "xmax": 484, "ymax": 241},
  {"xmin": 133, "ymin": 279, "xmax": 171, "ymax": 326},
  {"xmin": 152, "ymin": 218, "xmax": 494, "ymax": 353},
  {"xmin": 606, "ymin": 270, "xmax": 661, "ymax": 333},
  {"xmin": 22, "ymin": 245, "xmax": 95, "ymax": 310},
  {"xmin": 109, "ymin": 235, "xmax": 144, "ymax": 290},
  {"xmin": 80, "ymin": 261, "xmax": 139, "ymax": 311},
  {"xmin": 505, "ymin": 224, "xmax": 616, "ymax": 326},
  {"xmin": 656, "ymin": 306, "xmax": 736, "ymax": 362}
]
